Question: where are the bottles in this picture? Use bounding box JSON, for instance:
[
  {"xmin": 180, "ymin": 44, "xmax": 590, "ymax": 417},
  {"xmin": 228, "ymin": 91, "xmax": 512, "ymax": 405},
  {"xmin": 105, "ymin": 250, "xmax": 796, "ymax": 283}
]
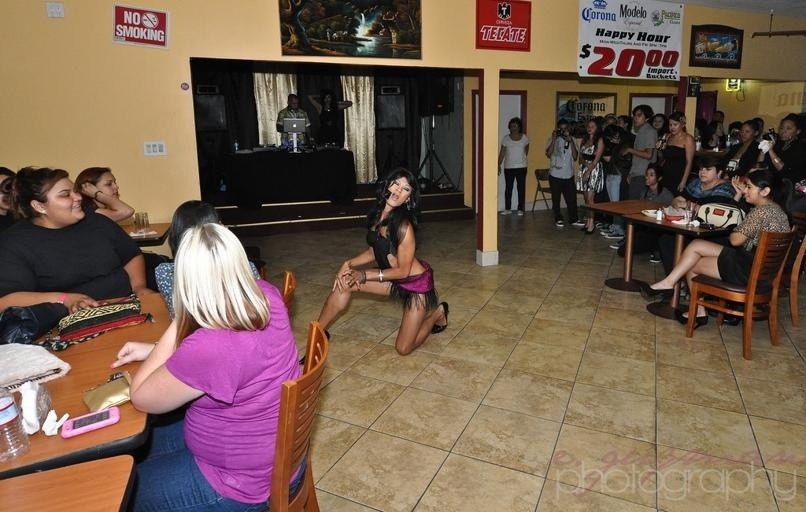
[
  {"xmin": 726, "ymin": 134, "xmax": 733, "ymax": 151},
  {"xmin": 696, "ymin": 134, "xmax": 703, "ymax": 152},
  {"xmin": 0, "ymin": 385, "xmax": 31, "ymax": 460},
  {"xmin": 656, "ymin": 134, "xmax": 666, "ymax": 150},
  {"xmin": 657, "ymin": 207, "xmax": 663, "ymax": 221}
]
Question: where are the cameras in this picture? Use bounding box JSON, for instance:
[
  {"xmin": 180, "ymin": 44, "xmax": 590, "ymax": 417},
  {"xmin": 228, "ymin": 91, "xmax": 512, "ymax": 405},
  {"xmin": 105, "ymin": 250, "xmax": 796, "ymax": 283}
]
[
  {"xmin": 556, "ymin": 128, "xmax": 562, "ymax": 137},
  {"xmin": 730, "ymin": 128, "xmax": 740, "ymax": 137}
]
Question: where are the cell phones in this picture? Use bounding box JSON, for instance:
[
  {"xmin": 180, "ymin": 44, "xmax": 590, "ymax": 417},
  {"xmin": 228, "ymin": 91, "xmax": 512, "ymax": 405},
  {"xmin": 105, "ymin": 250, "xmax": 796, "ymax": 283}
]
[{"xmin": 61, "ymin": 406, "xmax": 120, "ymax": 439}]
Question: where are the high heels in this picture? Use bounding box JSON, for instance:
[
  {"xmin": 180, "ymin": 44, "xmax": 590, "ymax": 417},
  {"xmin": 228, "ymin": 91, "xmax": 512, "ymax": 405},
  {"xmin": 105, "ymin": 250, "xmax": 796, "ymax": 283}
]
[
  {"xmin": 675, "ymin": 309, "xmax": 710, "ymax": 330},
  {"xmin": 297, "ymin": 329, "xmax": 331, "ymax": 365},
  {"xmin": 431, "ymin": 302, "xmax": 449, "ymax": 334},
  {"xmin": 578, "ymin": 225, "xmax": 588, "ymax": 232},
  {"xmin": 585, "ymin": 226, "xmax": 596, "ymax": 235},
  {"xmin": 639, "ymin": 282, "xmax": 675, "ymax": 303}
]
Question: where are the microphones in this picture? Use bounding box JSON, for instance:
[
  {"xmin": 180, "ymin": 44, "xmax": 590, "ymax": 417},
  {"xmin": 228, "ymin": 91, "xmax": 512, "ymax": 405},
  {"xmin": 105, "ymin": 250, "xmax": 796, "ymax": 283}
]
[{"xmin": 657, "ymin": 132, "xmax": 667, "ymax": 151}]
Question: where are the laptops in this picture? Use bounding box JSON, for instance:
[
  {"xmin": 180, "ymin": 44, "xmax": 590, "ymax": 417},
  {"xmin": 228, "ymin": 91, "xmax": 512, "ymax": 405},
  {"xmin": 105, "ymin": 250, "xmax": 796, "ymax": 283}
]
[{"xmin": 283, "ymin": 118, "xmax": 307, "ymax": 133}]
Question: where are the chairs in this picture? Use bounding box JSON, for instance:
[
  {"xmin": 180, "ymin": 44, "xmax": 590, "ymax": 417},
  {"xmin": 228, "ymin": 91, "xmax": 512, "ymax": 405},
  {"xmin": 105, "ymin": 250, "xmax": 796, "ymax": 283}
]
[
  {"xmin": 532, "ymin": 168, "xmax": 553, "ymax": 213},
  {"xmin": 685, "ymin": 224, "xmax": 799, "ymax": 360},
  {"xmin": 268, "ymin": 321, "xmax": 331, "ymax": 512},
  {"xmin": 281, "ymin": 270, "xmax": 295, "ymax": 317},
  {"xmin": 778, "ymin": 211, "xmax": 806, "ymax": 327}
]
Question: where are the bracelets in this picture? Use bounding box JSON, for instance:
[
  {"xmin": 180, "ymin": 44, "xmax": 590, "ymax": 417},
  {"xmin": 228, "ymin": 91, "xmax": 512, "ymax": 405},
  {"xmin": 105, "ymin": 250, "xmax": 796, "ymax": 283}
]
[
  {"xmin": 378, "ymin": 268, "xmax": 383, "ymax": 282},
  {"xmin": 309, "ymin": 137, "xmax": 314, "ymax": 140},
  {"xmin": 361, "ymin": 268, "xmax": 366, "ymax": 284}
]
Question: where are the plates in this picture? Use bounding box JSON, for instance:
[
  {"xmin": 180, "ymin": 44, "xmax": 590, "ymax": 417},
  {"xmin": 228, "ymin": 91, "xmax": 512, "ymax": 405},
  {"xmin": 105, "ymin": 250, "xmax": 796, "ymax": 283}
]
[
  {"xmin": 666, "ymin": 215, "xmax": 684, "ymax": 221},
  {"xmin": 641, "ymin": 209, "xmax": 664, "ymax": 218}
]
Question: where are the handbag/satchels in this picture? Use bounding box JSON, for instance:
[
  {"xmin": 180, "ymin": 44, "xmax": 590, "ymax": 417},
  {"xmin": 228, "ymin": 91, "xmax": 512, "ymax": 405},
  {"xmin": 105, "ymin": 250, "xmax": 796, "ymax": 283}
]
[
  {"xmin": 52, "ymin": 294, "xmax": 147, "ymax": 351},
  {"xmin": 697, "ymin": 202, "xmax": 746, "ymax": 231}
]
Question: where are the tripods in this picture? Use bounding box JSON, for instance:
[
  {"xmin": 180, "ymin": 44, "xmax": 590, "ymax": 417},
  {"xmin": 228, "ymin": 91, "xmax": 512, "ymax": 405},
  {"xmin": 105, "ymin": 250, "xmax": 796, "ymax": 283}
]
[{"xmin": 414, "ymin": 116, "xmax": 455, "ymax": 187}]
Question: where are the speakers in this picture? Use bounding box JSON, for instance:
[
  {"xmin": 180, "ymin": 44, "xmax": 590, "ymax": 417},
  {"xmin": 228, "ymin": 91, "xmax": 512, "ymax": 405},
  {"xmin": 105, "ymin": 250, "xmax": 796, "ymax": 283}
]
[{"xmin": 417, "ymin": 76, "xmax": 454, "ymax": 117}]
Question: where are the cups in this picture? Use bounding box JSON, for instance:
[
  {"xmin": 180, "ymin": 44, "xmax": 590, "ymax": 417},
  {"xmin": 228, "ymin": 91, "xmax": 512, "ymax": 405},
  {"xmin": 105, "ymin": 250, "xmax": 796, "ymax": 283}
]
[
  {"xmin": 686, "ymin": 207, "xmax": 695, "ymax": 227},
  {"xmin": 134, "ymin": 211, "xmax": 151, "ymax": 237}
]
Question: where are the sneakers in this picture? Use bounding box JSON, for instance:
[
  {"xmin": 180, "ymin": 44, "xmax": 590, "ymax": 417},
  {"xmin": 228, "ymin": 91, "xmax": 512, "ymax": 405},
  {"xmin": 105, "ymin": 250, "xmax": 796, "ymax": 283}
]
[
  {"xmin": 605, "ymin": 233, "xmax": 625, "ymax": 239},
  {"xmin": 649, "ymin": 256, "xmax": 662, "ymax": 263},
  {"xmin": 555, "ymin": 220, "xmax": 565, "ymax": 227},
  {"xmin": 517, "ymin": 211, "xmax": 524, "ymax": 216},
  {"xmin": 500, "ymin": 211, "xmax": 513, "ymax": 215},
  {"xmin": 600, "ymin": 231, "xmax": 612, "ymax": 236},
  {"xmin": 571, "ymin": 220, "xmax": 585, "ymax": 225},
  {"xmin": 609, "ymin": 244, "xmax": 620, "ymax": 250}
]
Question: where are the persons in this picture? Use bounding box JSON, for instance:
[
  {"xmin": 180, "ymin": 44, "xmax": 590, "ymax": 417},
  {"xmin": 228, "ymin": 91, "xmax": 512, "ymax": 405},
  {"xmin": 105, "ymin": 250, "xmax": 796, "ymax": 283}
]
[
  {"xmin": 498, "ymin": 117, "xmax": 531, "ymax": 216},
  {"xmin": 299, "ymin": 168, "xmax": 449, "ymax": 369},
  {"xmin": 309, "ymin": 87, "xmax": 353, "ymax": 150},
  {"xmin": 1, "ymin": 163, "xmax": 259, "ymax": 328},
  {"xmin": 275, "ymin": 92, "xmax": 314, "ymax": 148},
  {"xmin": 543, "ymin": 103, "xmax": 804, "ymax": 330},
  {"xmin": 109, "ymin": 221, "xmax": 307, "ymax": 511}
]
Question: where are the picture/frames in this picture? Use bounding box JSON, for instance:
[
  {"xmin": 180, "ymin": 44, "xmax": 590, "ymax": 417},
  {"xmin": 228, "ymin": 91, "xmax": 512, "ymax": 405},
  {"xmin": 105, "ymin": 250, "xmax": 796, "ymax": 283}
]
[{"xmin": 688, "ymin": 23, "xmax": 744, "ymax": 71}]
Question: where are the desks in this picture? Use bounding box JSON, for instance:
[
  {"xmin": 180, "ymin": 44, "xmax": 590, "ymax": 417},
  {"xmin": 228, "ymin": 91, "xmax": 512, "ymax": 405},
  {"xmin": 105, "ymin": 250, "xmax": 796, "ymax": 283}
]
[
  {"xmin": 0, "ymin": 292, "xmax": 183, "ymax": 478},
  {"xmin": 694, "ymin": 146, "xmax": 726, "ymax": 159},
  {"xmin": 622, "ymin": 211, "xmax": 727, "ymax": 322},
  {"xmin": 1, "ymin": 455, "xmax": 135, "ymax": 511},
  {"xmin": 580, "ymin": 199, "xmax": 668, "ymax": 292},
  {"xmin": 117, "ymin": 222, "xmax": 172, "ymax": 247},
  {"xmin": 231, "ymin": 145, "xmax": 356, "ymax": 215}
]
